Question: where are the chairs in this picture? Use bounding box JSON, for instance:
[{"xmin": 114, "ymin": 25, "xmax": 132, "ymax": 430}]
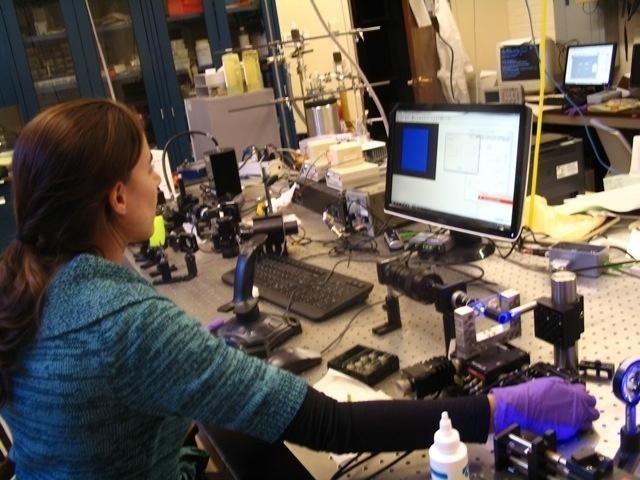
[{"xmin": 589, "ymin": 116, "xmax": 640, "ymax": 176}]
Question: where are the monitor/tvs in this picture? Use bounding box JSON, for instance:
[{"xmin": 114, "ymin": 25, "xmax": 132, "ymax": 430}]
[
  {"xmin": 384, "ymin": 104, "xmax": 533, "ymax": 262},
  {"xmin": 497, "ymin": 37, "xmax": 565, "ymax": 102},
  {"xmin": 562, "ymin": 43, "xmax": 619, "ymax": 90}
]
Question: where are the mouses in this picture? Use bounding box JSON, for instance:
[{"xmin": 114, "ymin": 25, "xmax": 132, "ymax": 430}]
[{"xmin": 267, "ymin": 348, "xmax": 321, "ymax": 376}]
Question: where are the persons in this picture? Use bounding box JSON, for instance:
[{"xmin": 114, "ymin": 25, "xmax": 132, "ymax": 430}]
[{"xmin": 0, "ymin": 99, "xmax": 600, "ymax": 480}]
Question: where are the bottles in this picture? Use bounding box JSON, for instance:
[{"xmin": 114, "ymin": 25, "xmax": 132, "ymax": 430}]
[
  {"xmin": 305, "ymin": 97, "xmax": 342, "ymax": 139},
  {"xmin": 193, "ymin": 27, "xmax": 264, "ymax": 96},
  {"xmin": 427, "ymin": 410, "xmax": 471, "ymax": 480}
]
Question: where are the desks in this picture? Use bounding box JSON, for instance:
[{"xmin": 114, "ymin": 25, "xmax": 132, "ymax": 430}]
[{"xmin": 512, "ymin": 99, "xmax": 640, "ymax": 187}]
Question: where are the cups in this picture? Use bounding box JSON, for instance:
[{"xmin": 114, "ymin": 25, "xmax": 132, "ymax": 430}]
[{"xmin": 32, "ymin": 7, "xmax": 48, "ymax": 36}]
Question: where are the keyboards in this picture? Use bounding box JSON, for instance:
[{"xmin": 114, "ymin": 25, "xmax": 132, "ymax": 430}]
[
  {"xmin": 221, "ymin": 251, "xmax": 373, "ymax": 321},
  {"xmin": 530, "ymin": 96, "xmax": 583, "ymax": 105}
]
